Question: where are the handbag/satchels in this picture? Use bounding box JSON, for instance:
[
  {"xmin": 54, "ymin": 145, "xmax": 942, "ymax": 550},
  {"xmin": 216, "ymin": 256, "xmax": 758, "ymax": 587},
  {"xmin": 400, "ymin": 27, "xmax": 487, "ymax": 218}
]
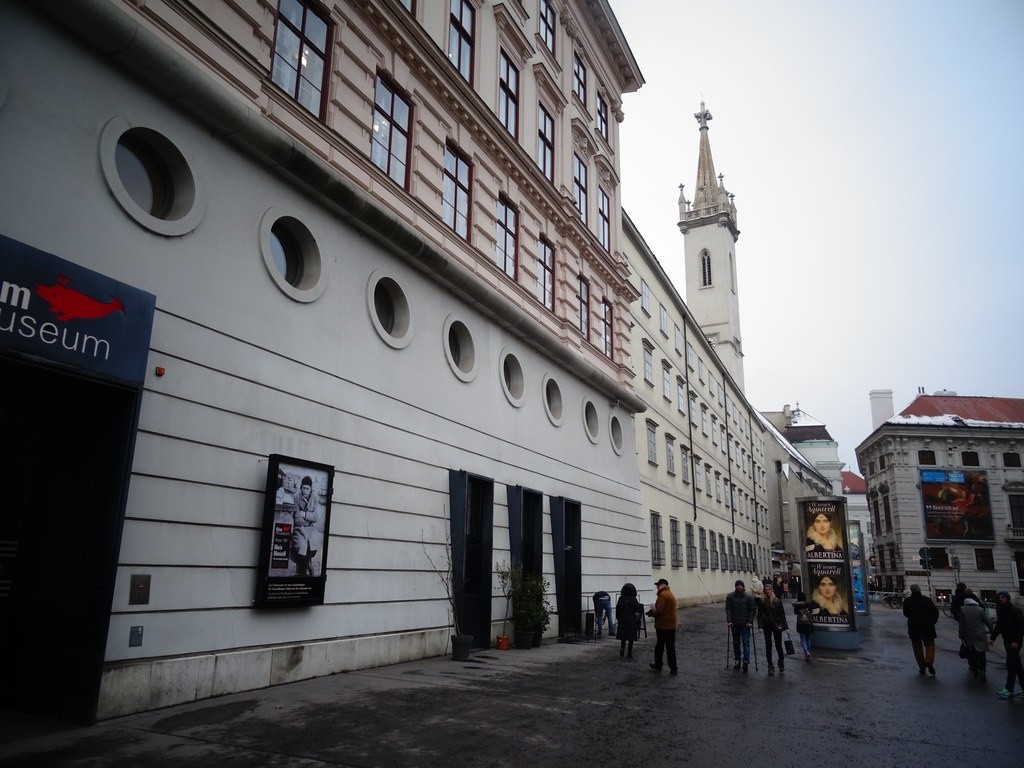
[
  {"xmin": 960, "ymin": 644, "xmax": 966, "ymax": 657},
  {"xmin": 785, "ymin": 640, "xmax": 795, "ymax": 654}
]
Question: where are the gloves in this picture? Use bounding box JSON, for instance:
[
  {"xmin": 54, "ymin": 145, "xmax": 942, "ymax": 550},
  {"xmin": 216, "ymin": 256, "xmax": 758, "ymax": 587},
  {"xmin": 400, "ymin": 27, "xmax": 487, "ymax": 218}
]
[{"xmin": 646, "ymin": 609, "xmax": 653, "ymax": 617}]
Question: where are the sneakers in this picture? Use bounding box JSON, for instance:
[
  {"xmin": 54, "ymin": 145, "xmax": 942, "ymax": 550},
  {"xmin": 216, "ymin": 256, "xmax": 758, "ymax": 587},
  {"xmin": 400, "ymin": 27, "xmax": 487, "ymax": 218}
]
[{"xmin": 996, "ymin": 686, "xmax": 1014, "ymax": 699}]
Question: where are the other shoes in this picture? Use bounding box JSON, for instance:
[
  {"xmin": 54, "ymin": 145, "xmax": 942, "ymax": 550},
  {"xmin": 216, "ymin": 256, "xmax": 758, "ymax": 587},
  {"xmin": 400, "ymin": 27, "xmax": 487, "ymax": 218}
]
[
  {"xmin": 628, "ymin": 654, "xmax": 633, "ymax": 657},
  {"xmin": 649, "ymin": 663, "xmax": 662, "ymax": 671},
  {"xmin": 609, "ymin": 632, "xmax": 616, "ymax": 636},
  {"xmin": 733, "ymin": 661, "xmax": 741, "ymax": 669},
  {"xmin": 620, "ymin": 648, "xmax": 625, "ymax": 657},
  {"xmin": 308, "ymin": 558, "xmax": 314, "ymax": 577},
  {"xmin": 768, "ymin": 665, "xmax": 775, "ymax": 675},
  {"xmin": 671, "ymin": 667, "xmax": 678, "ymax": 676},
  {"xmin": 779, "ymin": 664, "xmax": 784, "ymax": 672},
  {"xmin": 597, "ymin": 631, "xmax": 601, "ymax": 635},
  {"xmin": 919, "ymin": 670, "xmax": 925, "ymax": 675},
  {"xmin": 742, "ymin": 661, "xmax": 748, "ymax": 669},
  {"xmin": 926, "ymin": 660, "xmax": 935, "ymax": 675}
]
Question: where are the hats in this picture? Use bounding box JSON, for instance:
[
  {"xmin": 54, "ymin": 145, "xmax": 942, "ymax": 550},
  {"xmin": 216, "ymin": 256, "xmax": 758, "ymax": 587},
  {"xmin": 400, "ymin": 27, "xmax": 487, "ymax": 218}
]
[
  {"xmin": 997, "ymin": 589, "xmax": 1011, "ymax": 600},
  {"xmin": 964, "ymin": 598, "xmax": 979, "ymax": 606},
  {"xmin": 302, "ymin": 476, "xmax": 312, "ymax": 486},
  {"xmin": 655, "ymin": 578, "xmax": 668, "ymax": 586}
]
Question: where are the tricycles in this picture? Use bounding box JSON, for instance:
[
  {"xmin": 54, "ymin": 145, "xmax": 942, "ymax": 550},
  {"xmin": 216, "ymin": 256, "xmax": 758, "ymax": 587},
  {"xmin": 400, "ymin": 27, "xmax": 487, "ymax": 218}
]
[{"xmin": 886, "ymin": 593, "xmax": 906, "ymax": 609}]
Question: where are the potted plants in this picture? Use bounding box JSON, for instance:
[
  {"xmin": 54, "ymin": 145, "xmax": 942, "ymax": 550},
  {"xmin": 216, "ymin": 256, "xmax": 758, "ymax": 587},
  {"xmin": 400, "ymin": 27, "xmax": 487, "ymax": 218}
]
[
  {"xmin": 514, "ymin": 577, "xmax": 536, "ymax": 649},
  {"xmin": 529, "ymin": 579, "xmax": 549, "ymax": 646},
  {"xmin": 419, "ymin": 508, "xmax": 475, "ymax": 661},
  {"xmin": 495, "ymin": 564, "xmax": 520, "ymax": 649}
]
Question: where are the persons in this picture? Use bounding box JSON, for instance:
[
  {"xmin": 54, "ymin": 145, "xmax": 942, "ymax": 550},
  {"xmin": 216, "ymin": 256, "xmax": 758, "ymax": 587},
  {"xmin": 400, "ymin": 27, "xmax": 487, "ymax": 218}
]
[
  {"xmin": 616, "ymin": 583, "xmax": 642, "ymax": 658},
  {"xmin": 757, "ymin": 584, "xmax": 786, "ymax": 675},
  {"xmin": 958, "ymin": 594, "xmax": 993, "ymax": 677},
  {"xmin": 751, "ymin": 575, "xmax": 801, "ymax": 601},
  {"xmin": 725, "ymin": 580, "xmax": 756, "ymax": 670},
  {"xmin": 649, "ymin": 579, "xmax": 678, "ymax": 675},
  {"xmin": 950, "ymin": 583, "xmax": 986, "ymax": 622},
  {"xmin": 810, "ymin": 574, "xmax": 847, "ymax": 616},
  {"xmin": 792, "ymin": 592, "xmax": 814, "ymax": 656},
  {"xmin": 806, "ymin": 512, "xmax": 842, "ymax": 560},
  {"xmin": 988, "ymin": 591, "xmax": 1024, "ymax": 698},
  {"xmin": 292, "ymin": 475, "xmax": 320, "ymax": 575},
  {"xmin": 870, "ymin": 582, "xmax": 891, "ymax": 596},
  {"xmin": 593, "ymin": 591, "xmax": 616, "ymax": 636},
  {"xmin": 903, "ymin": 584, "xmax": 939, "ymax": 675}
]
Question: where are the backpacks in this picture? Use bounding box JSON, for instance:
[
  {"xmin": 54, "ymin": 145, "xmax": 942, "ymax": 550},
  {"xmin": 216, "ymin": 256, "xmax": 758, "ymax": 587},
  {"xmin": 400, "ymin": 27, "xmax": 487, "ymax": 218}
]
[{"xmin": 798, "ymin": 608, "xmax": 810, "ymax": 625}]
